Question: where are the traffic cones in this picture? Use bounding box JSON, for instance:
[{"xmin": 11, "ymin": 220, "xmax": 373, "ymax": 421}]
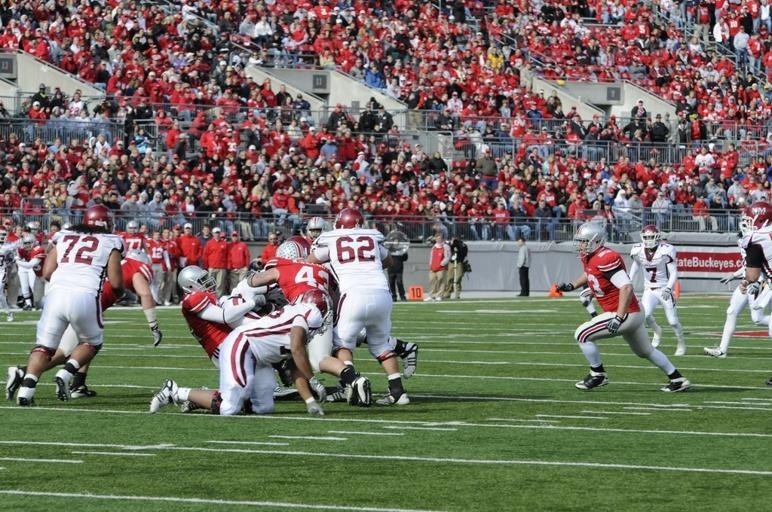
[{"xmin": 549, "ymin": 282, "xmax": 562, "ymax": 298}]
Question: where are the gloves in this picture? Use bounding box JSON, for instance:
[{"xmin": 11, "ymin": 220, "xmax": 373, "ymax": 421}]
[
  {"xmin": 149, "ymin": 323, "xmax": 162, "ymax": 346},
  {"xmin": 747, "ymin": 282, "xmax": 760, "ymax": 295},
  {"xmin": 556, "ymin": 282, "xmax": 574, "ymax": 292},
  {"xmin": 607, "ymin": 315, "xmax": 623, "ymax": 336},
  {"xmin": 719, "ymin": 273, "xmax": 734, "ymax": 284},
  {"xmin": 305, "ymin": 400, "xmax": 325, "ymax": 416},
  {"xmin": 660, "ymin": 287, "xmax": 672, "ymax": 301},
  {"xmin": 262, "ymin": 287, "xmax": 284, "ymax": 305},
  {"xmin": 119, "ymin": 289, "xmax": 138, "ymax": 302}
]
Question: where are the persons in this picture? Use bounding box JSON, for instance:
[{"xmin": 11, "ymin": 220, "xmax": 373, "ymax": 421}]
[
  {"xmin": 627, "ymin": 225, "xmax": 687, "ymax": 357},
  {"xmin": 0, "ymin": 0, "xmax": 772, "ymax": 317},
  {"xmin": 555, "ymin": 223, "xmax": 690, "ymax": 393},
  {"xmin": 704, "ymin": 203, "xmax": 772, "ymax": 385},
  {"xmin": 6, "ymin": 249, "xmax": 161, "ymax": 402},
  {"xmin": 17, "ymin": 204, "xmax": 122, "ymax": 406},
  {"xmin": 148, "ymin": 207, "xmax": 419, "ymax": 415}
]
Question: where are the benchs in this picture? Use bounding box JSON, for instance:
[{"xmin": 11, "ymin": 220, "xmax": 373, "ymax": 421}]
[{"xmin": 646, "ymin": 211, "xmax": 696, "ymax": 223}]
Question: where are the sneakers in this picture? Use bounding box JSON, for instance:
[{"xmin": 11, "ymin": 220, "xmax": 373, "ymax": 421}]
[
  {"xmin": 6, "ymin": 365, "xmax": 96, "ymax": 405},
  {"xmin": 149, "ymin": 378, "xmax": 179, "ymax": 413},
  {"xmin": 674, "ymin": 344, "xmax": 686, "ymax": 356},
  {"xmin": 574, "ymin": 369, "xmax": 609, "ymax": 390},
  {"xmin": 273, "ymin": 341, "xmax": 419, "ymax": 405},
  {"xmin": 651, "ymin": 326, "xmax": 662, "ymax": 348},
  {"xmin": 7, "ymin": 311, "xmax": 14, "ymax": 321},
  {"xmin": 163, "ymin": 299, "xmax": 171, "ymax": 306},
  {"xmin": 659, "ymin": 377, "xmax": 690, "ymax": 392},
  {"xmin": 180, "ymin": 384, "xmax": 210, "ymax": 412},
  {"xmin": 703, "ymin": 346, "xmax": 727, "ymax": 359},
  {"xmin": 23, "ymin": 304, "xmax": 33, "ymax": 311}
]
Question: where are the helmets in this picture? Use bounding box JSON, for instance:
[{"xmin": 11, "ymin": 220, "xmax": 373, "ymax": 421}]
[
  {"xmin": 274, "ymin": 207, "xmax": 364, "ymax": 259},
  {"xmin": 125, "ymin": 249, "xmax": 152, "ymax": 265},
  {"xmin": 126, "ymin": 221, "xmax": 141, "ymax": 234},
  {"xmin": 301, "ymin": 288, "xmax": 334, "ymax": 334},
  {"xmin": 738, "ymin": 202, "xmax": 771, "ymax": 238},
  {"xmin": 2, "ymin": 217, "xmax": 45, "ymax": 254},
  {"xmin": 176, "ymin": 265, "xmax": 217, "ymax": 295},
  {"xmin": 82, "ymin": 205, "xmax": 115, "ymax": 233},
  {"xmin": 574, "ymin": 222, "xmax": 607, "ymax": 260},
  {"xmin": 639, "ymin": 224, "xmax": 660, "ymax": 249}
]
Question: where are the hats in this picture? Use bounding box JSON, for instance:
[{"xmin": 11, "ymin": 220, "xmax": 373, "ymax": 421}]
[
  {"xmin": 0, "ymin": 1, "xmax": 771, "ymax": 212},
  {"xmin": 174, "ymin": 223, "xmax": 238, "ymax": 238}
]
